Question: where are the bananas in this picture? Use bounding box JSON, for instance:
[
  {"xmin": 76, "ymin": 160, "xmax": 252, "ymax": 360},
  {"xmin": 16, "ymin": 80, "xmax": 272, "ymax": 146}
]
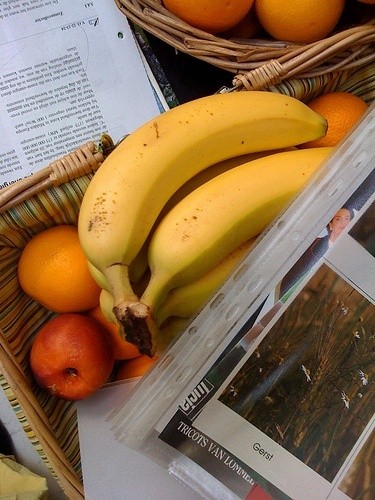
[{"xmin": 78, "ymin": 91, "xmax": 336, "ymax": 359}]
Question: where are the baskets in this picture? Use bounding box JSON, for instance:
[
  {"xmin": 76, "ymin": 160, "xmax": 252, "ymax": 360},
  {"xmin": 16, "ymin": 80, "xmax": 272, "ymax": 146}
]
[
  {"xmin": 0, "ymin": 26, "xmax": 374, "ymax": 499},
  {"xmin": 114, "ymin": 0, "xmax": 375, "ymax": 76}
]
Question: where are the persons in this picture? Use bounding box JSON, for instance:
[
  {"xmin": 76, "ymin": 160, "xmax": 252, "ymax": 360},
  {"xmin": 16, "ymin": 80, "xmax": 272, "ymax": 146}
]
[{"xmin": 279, "ymin": 206, "xmax": 355, "ymax": 300}]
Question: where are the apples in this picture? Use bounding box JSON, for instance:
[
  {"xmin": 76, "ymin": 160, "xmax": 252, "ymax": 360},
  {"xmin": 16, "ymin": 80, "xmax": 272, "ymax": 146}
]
[{"xmin": 31, "ymin": 315, "xmax": 111, "ymax": 401}]
